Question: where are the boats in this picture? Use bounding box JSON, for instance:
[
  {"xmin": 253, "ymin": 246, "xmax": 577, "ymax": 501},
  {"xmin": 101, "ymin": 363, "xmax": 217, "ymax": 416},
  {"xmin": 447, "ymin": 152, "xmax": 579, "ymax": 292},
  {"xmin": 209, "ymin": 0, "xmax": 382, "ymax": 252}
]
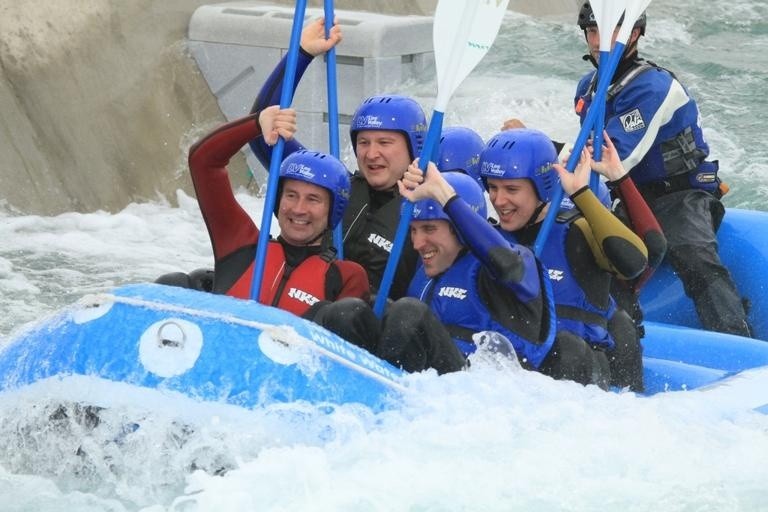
[{"xmin": 0, "ymin": 208, "xmax": 768, "ymax": 413}]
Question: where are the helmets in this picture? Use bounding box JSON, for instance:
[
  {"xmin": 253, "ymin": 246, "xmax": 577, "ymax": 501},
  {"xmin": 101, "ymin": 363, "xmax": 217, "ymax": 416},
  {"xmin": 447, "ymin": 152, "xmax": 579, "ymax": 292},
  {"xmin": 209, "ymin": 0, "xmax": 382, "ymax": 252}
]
[
  {"xmin": 578, "ymin": 0, "xmax": 647, "ymax": 38},
  {"xmin": 274, "ymin": 151, "xmax": 351, "ymax": 231},
  {"xmin": 478, "ymin": 127, "xmax": 558, "ymax": 204},
  {"xmin": 412, "ymin": 171, "xmax": 487, "ymax": 247},
  {"xmin": 349, "ymin": 96, "xmax": 427, "ymax": 161},
  {"xmin": 439, "ymin": 126, "xmax": 487, "ymax": 192}
]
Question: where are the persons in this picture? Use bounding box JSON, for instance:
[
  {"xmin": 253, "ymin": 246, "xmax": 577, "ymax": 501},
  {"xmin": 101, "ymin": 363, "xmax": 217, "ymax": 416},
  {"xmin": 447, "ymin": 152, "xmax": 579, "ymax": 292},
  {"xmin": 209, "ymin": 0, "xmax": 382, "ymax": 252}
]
[
  {"xmin": 500, "ymin": 0, "xmax": 755, "ymax": 342},
  {"xmin": 187, "ymin": 13, "xmax": 427, "ymax": 298},
  {"xmin": 155, "ymin": 96, "xmax": 667, "ymax": 393}
]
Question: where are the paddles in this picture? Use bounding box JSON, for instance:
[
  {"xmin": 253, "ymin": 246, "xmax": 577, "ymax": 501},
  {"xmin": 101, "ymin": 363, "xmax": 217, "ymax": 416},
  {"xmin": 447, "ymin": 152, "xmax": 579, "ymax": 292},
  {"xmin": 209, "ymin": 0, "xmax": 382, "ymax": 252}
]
[
  {"xmin": 584, "ymin": 0, "xmax": 626, "ymax": 196},
  {"xmin": 532, "ymin": 0, "xmax": 652, "ymax": 259},
  {"xmin": 369, "ymin": 0, "xmax": 509, "ymax": 317}
]
